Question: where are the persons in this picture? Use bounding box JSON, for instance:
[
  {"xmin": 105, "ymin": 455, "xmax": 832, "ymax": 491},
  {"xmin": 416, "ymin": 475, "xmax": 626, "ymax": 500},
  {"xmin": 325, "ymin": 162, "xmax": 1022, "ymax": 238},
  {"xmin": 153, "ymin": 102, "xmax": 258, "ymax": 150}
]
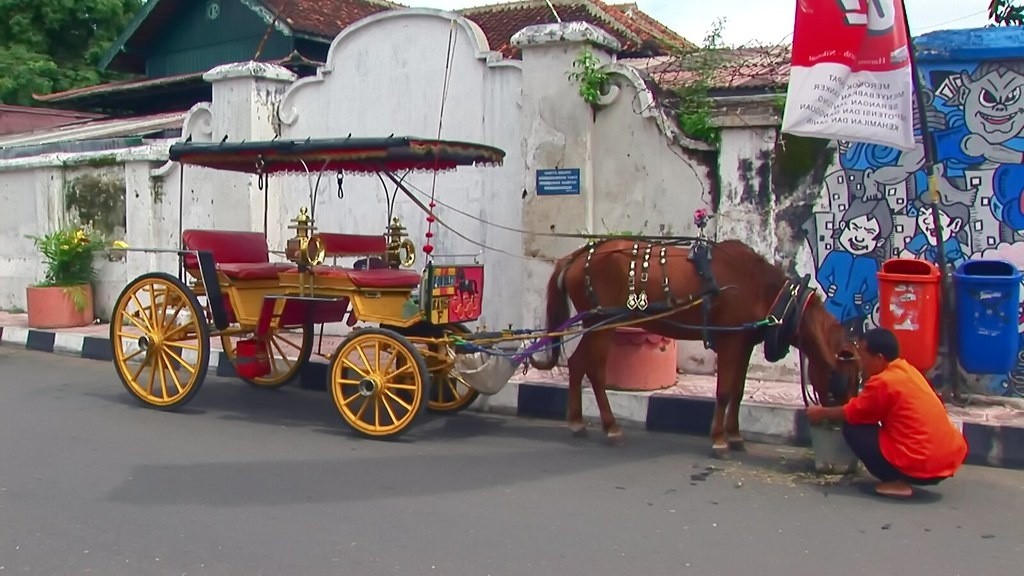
[{"xmin": 807, "ymin": 328, "xmax": 967, "ymax": 497}]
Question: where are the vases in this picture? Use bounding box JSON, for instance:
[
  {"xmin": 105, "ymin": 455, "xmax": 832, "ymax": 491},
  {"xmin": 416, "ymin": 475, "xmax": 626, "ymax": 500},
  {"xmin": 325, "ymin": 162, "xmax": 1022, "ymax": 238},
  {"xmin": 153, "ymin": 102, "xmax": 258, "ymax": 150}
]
[
  {"xmin": 27, "ymin": 283, "xmax": 94, "ymax": 329},
  {"xmin": 605, "ymin": 326, "xmax": 677, "ymax": 390}
]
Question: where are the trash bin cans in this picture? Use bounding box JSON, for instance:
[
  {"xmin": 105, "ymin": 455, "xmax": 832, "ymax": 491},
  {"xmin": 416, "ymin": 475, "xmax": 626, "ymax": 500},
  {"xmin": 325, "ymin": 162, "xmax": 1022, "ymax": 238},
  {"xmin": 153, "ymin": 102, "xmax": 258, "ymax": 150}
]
[
  {"xmin": 954, "ymin": 260, "xmax": 1021, "ymax": 373},
  {"xmin": 877, "ymin": 259, "xmax": 942, "ymax": 372}
]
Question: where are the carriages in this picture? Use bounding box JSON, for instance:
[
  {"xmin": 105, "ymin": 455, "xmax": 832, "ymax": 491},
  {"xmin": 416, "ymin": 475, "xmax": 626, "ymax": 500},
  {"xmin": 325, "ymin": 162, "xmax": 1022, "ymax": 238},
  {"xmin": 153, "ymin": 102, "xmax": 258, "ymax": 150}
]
[{"xmin": 109, "ymin": 135, "xmax": 866, "ymax": 462}]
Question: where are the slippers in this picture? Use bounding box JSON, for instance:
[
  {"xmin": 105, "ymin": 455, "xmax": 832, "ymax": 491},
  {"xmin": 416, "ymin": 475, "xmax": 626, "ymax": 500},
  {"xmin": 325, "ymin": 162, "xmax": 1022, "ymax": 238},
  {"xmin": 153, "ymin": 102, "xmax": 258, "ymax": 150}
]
[{"xmin": 864, "ymin": 481, "xmax": 914, "ymax": 500}]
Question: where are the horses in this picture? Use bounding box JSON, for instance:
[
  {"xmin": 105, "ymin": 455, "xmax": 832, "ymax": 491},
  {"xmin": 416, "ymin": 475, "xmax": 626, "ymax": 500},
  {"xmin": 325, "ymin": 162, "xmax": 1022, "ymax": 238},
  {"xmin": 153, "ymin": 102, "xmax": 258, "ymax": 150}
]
[{"xmin": 529, "ymin": 238, "xmax": 864, "ymax": 461}]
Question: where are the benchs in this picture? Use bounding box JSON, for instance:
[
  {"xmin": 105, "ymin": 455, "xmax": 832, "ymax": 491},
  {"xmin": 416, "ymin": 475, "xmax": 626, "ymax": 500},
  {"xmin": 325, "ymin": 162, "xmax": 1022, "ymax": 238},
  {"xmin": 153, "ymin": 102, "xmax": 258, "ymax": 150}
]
[
  {"xmin": 277, "ymin": 233, "xmax": 421, "ymax": 288},
  {"xmin": 183, "ymin": 229, "xmax": 297, "ymax": 279}
]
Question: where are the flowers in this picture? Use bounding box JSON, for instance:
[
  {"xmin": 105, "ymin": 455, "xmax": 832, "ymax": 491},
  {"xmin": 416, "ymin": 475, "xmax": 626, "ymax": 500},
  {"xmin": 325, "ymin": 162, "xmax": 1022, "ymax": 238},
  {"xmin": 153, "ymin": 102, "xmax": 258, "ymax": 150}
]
[{"xmin": 24, "ymin": 230, "xmax": 100, "ymax": 314}]
